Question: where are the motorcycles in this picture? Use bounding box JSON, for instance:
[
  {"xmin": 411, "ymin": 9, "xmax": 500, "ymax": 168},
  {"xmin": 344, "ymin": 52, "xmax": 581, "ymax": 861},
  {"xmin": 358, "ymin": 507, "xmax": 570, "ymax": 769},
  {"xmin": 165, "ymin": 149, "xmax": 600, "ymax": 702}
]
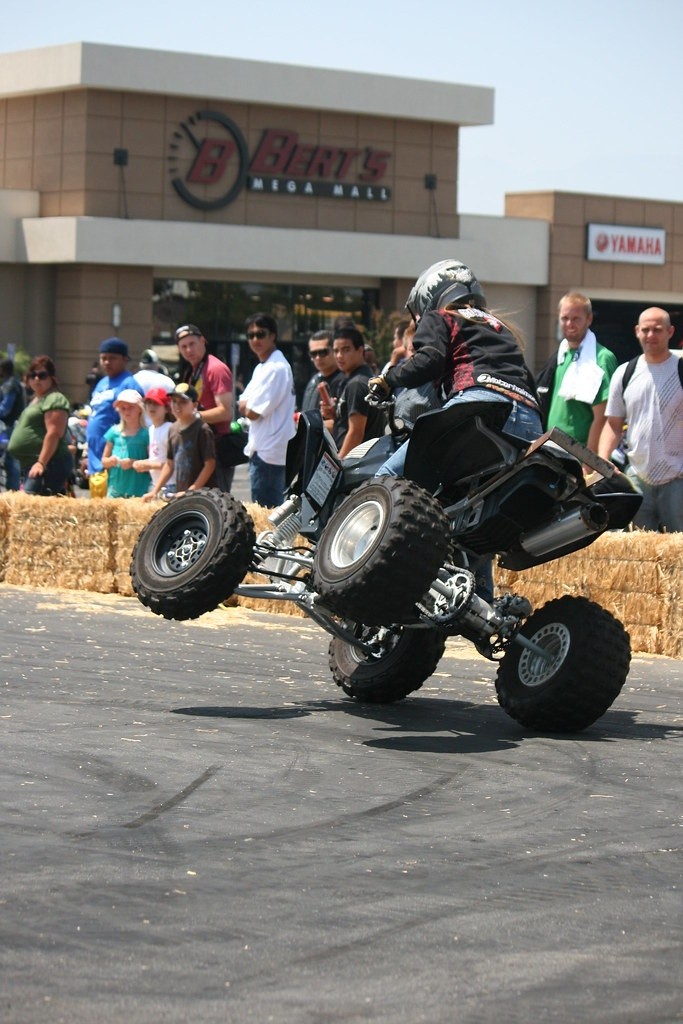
[{"xmin": 128, "ymin": 363, "xmax": 644, "ymax": 733}]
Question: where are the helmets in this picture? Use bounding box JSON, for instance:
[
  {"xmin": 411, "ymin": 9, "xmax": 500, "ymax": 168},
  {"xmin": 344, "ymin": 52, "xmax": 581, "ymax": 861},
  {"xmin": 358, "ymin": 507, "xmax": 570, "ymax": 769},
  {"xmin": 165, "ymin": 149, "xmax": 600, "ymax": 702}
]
[{"xmin": 405, "ymin": 260, "xmax": 486, "ymax": 321}]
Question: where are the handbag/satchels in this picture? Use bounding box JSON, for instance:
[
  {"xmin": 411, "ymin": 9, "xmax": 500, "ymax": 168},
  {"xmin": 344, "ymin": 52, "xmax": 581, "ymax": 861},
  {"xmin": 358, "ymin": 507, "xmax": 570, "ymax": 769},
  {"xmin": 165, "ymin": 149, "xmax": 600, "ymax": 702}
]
[{"xmin": 215, "ymin": 432, "xmax": 251, "ymax": 469}]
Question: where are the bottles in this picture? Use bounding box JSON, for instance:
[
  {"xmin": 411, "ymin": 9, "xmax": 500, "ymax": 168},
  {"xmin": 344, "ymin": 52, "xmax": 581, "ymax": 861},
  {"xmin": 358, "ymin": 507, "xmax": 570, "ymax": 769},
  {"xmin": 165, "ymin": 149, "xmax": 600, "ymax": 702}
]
[
  {"xmin": 230, "ymin": 416, "xmax": 245, "ymax": 433},
  {"xmin": 157, "ymin": 487, "xmax": 168, "ymax": 499}
]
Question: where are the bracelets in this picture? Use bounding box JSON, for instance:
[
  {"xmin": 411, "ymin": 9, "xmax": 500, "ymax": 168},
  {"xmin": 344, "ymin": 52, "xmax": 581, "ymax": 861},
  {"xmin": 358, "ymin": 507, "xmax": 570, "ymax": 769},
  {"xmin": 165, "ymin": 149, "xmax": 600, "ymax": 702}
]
[
  {"xmin": 194, "ymin": 411, "xmax": 202, "ymax": 420},
  {"xmin": 36, "ymin": 460, "xmax": 47, "ymax": 470}
]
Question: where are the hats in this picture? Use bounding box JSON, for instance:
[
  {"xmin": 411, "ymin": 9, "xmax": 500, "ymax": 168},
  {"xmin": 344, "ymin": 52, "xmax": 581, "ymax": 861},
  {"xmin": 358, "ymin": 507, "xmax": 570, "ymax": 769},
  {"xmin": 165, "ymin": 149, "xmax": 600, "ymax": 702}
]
[
  {"xmin": 144, "ymin": 388, "xmax": 171, "ymax": 406},
  {"xmin": 166, "ymin": 382, "xmax": 199, "ymax": 404},
  {"xmin": 174, "ymin": 325, "xmax": 202, "ymax": 343},
  {"xmin": 141, "ymin": 348, "xmax": 158, "ymax": 363},
  {"xmin": 99, "ymin": 338, "xmax": 131, "ymax": 361},
  {"xmin": 112, "ymin": 389, "xmax": 143, "ymax": 411}
]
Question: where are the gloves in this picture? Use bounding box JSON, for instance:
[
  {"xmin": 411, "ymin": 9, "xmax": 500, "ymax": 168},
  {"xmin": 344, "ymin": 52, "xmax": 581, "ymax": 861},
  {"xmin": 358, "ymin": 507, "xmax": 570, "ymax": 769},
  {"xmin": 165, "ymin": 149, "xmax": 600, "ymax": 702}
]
[{"xmin": 368, "ymin": 375, "xmax": 395, "ymax": 406}]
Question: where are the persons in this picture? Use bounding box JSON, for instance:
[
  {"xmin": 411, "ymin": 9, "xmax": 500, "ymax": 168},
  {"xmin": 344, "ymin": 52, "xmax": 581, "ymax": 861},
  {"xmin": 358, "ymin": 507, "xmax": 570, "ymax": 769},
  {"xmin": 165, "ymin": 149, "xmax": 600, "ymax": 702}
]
[
  {"xmin": 598, "ymin": 307, "xmax": 683, "ymax": 533},
  {"xmin": 0, "ymin": 291, "xmax": 683, "ymax": 533},
  {"xmin": 236, "ymin": 311, "xmax": 297, "ymax": 510},
  {"xmin": 6, "ymin": 354, "xmax": 74, "ymax": 497},
  {"xmin": 364, "ymin": 258, "xmax": 544, "ymax": 606},
  {"xmin": 174, "ymin": 325, "xmax": 234, "ymax": 494},
  {"xmin": 546, "ymin": 292, "xmax": 617, "ymax": 476},
  {"xmin": 79, "ymin": 337, "xmax": 145, "ymax": 479}
]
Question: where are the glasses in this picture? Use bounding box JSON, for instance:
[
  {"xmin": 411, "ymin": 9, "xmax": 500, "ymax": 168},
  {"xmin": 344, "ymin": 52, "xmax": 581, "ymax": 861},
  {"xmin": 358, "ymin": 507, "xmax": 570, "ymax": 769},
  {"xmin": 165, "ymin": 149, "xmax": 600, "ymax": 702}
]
[
  {"xmin": 309, "ymin": 349, "xmax": 332, "ymax": 358},
  {"xmin": 248, "ymin": 331, "xmax": 270, "ymax": 340},
  {"xmin": 30, "ymin": 371, "xmax": 50, "ymax": 380}
]
